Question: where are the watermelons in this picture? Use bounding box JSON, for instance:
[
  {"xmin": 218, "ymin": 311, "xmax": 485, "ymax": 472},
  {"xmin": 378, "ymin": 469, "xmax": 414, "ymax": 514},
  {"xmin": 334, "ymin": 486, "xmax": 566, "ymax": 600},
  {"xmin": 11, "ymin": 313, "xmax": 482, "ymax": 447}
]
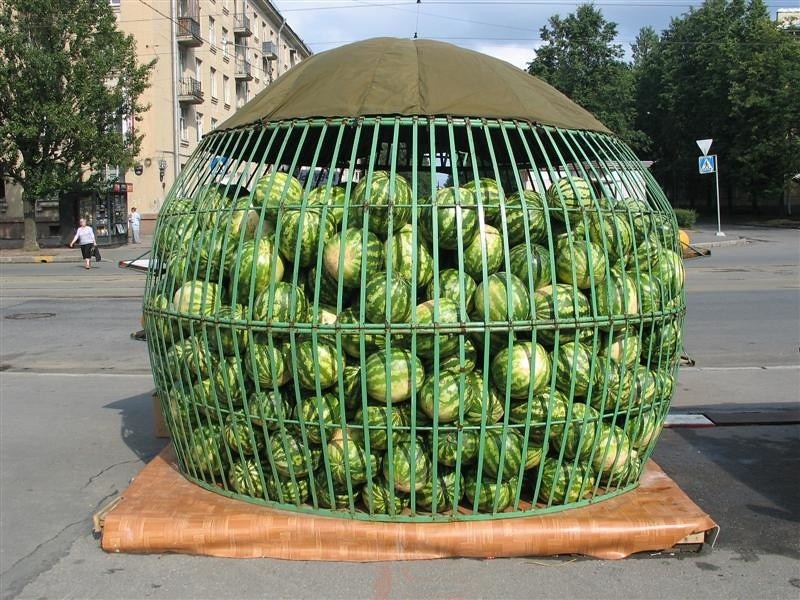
[{"xmin": 144, "ymin": 169, "xmax": 685, "ymax": 517}]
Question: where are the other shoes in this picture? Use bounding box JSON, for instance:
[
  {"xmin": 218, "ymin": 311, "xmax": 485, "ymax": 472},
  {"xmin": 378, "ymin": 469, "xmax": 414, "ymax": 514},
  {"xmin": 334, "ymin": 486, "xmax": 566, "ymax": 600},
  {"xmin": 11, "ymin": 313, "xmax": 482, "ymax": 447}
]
[{"xmin": 86, "ymin": 267, "xmax": 90, "ymax": 269}]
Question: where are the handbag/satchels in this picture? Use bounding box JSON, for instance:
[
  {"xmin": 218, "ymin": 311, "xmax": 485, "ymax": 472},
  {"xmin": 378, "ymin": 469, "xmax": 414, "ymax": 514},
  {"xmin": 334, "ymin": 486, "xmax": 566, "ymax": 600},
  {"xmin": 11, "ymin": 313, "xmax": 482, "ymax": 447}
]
[
  {"xmin": 91, "ymin": 248, "xmax": 95, "ymax": 256},
  {"xmin": 94, "ymin": 246, "xmax": 101, "ymax": 262}
]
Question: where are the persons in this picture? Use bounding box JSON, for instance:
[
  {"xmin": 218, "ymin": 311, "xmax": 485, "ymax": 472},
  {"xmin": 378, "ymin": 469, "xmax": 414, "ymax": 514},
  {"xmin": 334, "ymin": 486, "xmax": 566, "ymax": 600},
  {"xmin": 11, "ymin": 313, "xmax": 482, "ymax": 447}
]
[
  {"xmin": 70, "ymin": 218, "xmax": 97, "ymax": 270},
  {"xmin": 129, "ymin": 208, "xmax": 142, "ymax": 244}
]
